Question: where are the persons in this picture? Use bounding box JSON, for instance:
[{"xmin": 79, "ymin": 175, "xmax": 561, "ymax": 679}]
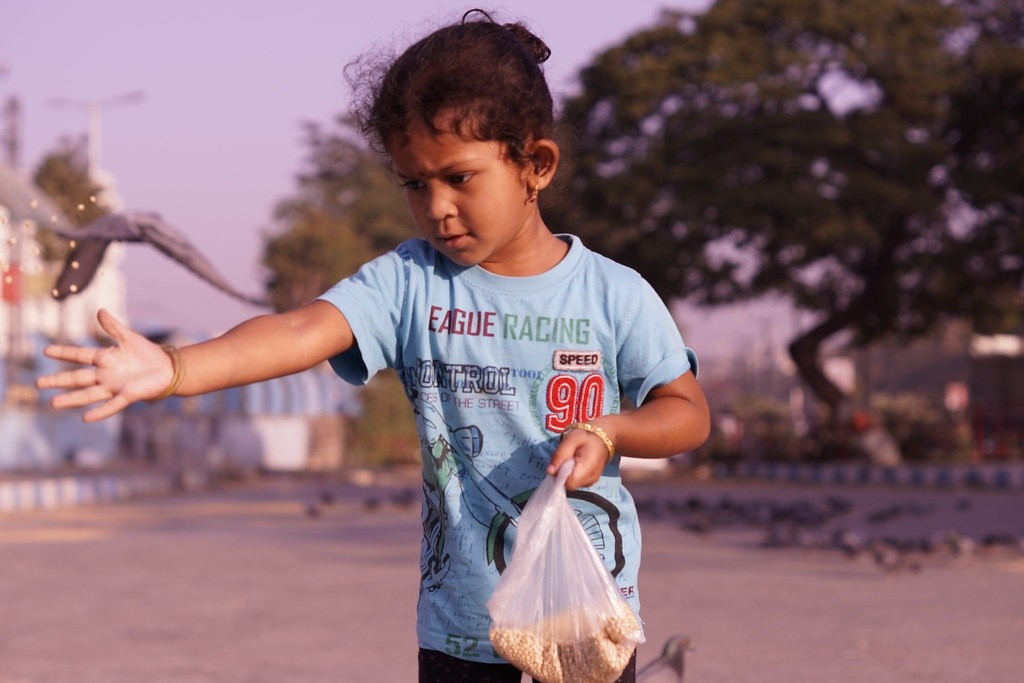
[{"xmin": 36, "ymin": 14, "xmax": 712, "ymax": 683}]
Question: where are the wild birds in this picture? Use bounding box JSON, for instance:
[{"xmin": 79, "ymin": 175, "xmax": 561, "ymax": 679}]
[
  {"xmin": 639, "ymin": 634, "xmax": 696, "ymax": 683},
  {"xmin": 50, "ymin": 205, "xmax": 278, "ymax": 307}
]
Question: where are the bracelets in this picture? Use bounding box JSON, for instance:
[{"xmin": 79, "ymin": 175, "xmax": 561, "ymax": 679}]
[
  {"xmin": 147, "ymin": 343, "xmax": 186, "ymax": 403},
  {"xmin": 558, "ymin": 421, "xmax": 613, "ymax": 463}
]
